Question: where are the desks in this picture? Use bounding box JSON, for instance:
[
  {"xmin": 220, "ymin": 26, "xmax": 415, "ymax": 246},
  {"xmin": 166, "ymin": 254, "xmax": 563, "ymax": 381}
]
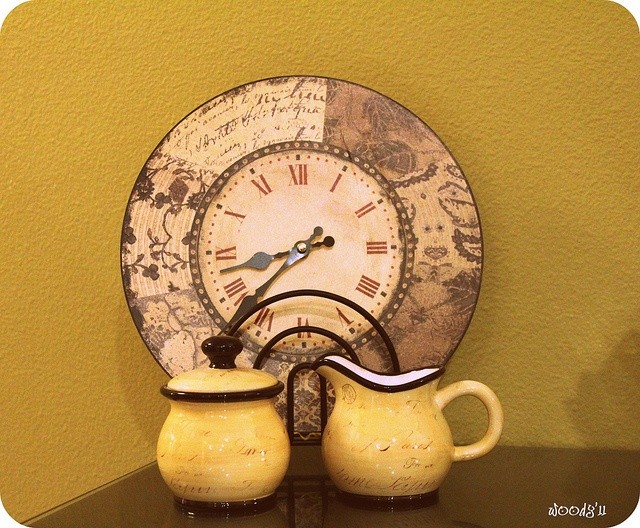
[{"xmin": 22, "ymin": 440, "xmax": 638, "ymax": 528}]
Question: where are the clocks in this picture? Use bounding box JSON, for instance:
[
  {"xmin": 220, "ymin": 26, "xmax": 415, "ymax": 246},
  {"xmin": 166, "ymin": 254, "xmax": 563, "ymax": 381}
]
[{"xmin": 118, "ymin": 72, "xmax": 486, "ymax": 447}]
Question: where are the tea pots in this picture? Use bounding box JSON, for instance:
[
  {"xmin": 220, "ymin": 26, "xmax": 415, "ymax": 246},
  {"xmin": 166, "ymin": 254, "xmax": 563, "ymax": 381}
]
[{"xmin": 310, "ymin": 353, "xmax": 503, "ymax": 511}]
